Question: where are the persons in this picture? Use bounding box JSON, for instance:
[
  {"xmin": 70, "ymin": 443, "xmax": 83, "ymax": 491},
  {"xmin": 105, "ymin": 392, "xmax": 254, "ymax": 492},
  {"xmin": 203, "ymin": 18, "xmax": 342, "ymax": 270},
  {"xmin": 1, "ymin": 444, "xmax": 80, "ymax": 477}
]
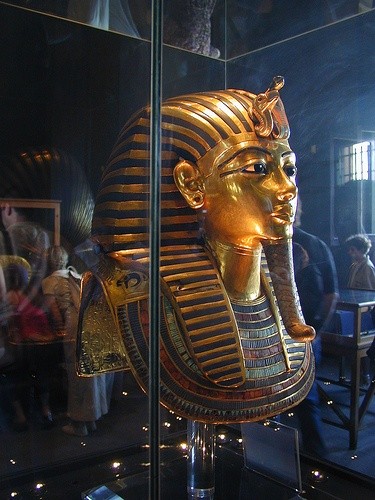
[
  {"xmin": 75, "ymin": 75, "xmax": 318, "ymax": 423},
  {"xmin": 2, "ymin": 191, "xmax": 375, "ymax": 461}
]
